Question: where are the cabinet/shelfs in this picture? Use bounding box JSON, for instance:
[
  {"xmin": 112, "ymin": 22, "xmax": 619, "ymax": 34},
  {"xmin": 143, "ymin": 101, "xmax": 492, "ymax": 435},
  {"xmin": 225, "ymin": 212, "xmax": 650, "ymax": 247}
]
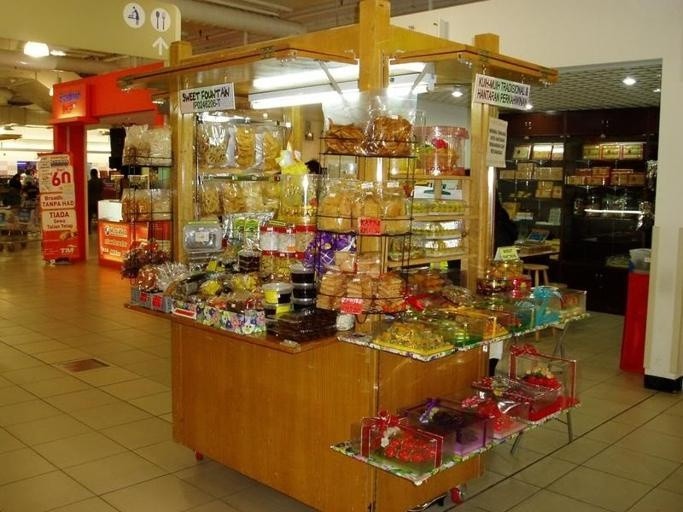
[
  {"xmin": 116, "ymin": 0, "xmax": 591, "ymax": 511},
  {"xmin": 495, "ymin": 104, "xmax": 659, "ymax": 312},
  {"xmin": 0, "ymin": 173, "xmax": 11, "ymax": 203},
  {"xmin": 327, "ymin": 293, "xmax": 591, "ymax": 483},
  {"xmin": 117, "ymin": 295, "xmax": 489, "ymax": 512}
]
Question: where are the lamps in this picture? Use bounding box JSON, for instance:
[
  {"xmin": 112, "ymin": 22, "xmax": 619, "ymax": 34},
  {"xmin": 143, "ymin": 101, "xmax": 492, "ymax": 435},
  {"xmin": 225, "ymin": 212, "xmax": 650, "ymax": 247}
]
[{"xmin": 248, "ymin": 62, "xmax": 436, "ymax": 112}]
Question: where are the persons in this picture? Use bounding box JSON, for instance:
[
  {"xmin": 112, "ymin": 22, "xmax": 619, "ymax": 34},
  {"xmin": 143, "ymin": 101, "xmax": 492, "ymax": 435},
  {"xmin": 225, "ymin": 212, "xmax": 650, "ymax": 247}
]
[
  {"xmin": 120, "ymin": 175, "xmax": 129, "ymax": 195},
  {"xmin": 3, "ymin": 168, "xmax": 39, "ymax": 206},
  {"xmin": 88, "ymin": 169, "xmax": 104, "ymax": 234}
]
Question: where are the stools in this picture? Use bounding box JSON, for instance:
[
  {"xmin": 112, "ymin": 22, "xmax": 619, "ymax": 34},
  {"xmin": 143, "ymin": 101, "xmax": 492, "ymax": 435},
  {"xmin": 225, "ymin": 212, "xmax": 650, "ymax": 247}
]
[{"xmin": 521, "ymin": 263, "xmax": 556, "ymax": 344}]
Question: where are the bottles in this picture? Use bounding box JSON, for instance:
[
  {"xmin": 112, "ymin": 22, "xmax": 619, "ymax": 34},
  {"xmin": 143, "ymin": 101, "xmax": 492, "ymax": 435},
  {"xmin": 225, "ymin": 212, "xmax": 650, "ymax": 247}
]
[
  {"xmin": 258, "ymin": 223, "xmax": 318, "ymax": 281},
  {"xmin": 572, "ymin": 190, "xmax": 638, "ymax": 218}
]
